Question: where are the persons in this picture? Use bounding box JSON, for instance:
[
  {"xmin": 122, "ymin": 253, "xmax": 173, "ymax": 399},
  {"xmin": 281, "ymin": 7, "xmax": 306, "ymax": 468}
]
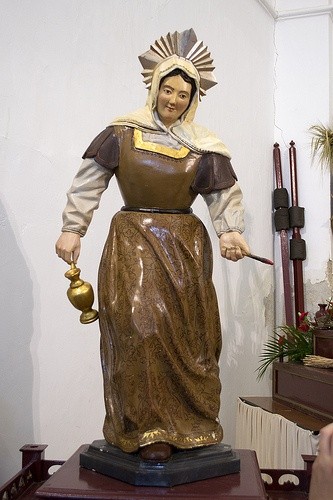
[{"xmin": 55, "ymin": 28, "xmax": 252, "ymax": 461}]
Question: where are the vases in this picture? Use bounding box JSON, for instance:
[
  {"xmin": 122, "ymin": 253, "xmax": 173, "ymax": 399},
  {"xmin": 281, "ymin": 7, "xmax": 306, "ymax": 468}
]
[{"xmin": 314, "ymin": 303, "xmax": 330, "ymax": 329}]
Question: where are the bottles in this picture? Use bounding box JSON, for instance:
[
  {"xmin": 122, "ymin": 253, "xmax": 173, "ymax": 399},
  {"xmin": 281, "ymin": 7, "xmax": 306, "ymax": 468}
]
[{"xmin": 315, "ymin": 304, "xmax": 329, "ymax": 329}]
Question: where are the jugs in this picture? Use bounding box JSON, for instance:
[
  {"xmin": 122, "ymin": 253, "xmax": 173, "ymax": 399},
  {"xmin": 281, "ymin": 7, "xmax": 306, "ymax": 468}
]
[{"xmin": 65, "ymin": 260, "xmax": 99, "ymax": 324}]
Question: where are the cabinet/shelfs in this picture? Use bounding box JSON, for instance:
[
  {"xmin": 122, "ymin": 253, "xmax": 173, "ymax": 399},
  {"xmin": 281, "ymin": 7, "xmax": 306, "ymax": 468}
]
[{"xmin": 238, "ymin": 329, "xmax": 333, "ymax": 434}]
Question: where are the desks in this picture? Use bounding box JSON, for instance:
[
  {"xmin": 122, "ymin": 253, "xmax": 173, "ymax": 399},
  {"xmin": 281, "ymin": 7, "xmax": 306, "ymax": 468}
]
[{"xmin": 0, "ymin": 445, "xmax": 317, "ymax": 500}]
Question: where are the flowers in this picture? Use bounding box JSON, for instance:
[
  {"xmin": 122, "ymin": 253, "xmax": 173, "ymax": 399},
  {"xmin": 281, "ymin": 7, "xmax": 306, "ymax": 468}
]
[{"xmin": 253, "ymin": 309, "xmax": 333, "ymax": 381}]
